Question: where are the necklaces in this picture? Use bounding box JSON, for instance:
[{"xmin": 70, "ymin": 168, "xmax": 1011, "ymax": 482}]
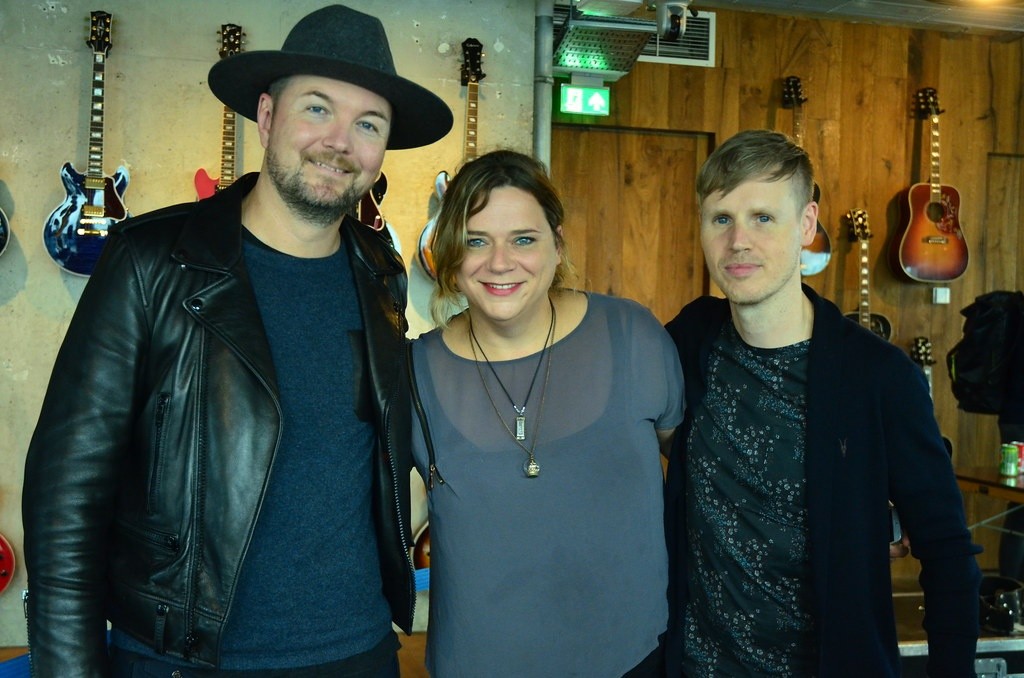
[{"xmin": 469, "ymin": 295, "xmax": 556, "ymax": 477}]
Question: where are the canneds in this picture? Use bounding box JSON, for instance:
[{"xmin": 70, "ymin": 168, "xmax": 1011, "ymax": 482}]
[{"xmin": 999, "ymin": 441, "xmax": 1024, "ymax": 477}]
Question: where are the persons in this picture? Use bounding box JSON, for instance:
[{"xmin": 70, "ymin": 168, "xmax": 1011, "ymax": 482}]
[
  {"xmin": 665, "ymin": 129, "xmax": 984, "ymax": 678},
  {"xmin": 998, "ymin": 290, "xmax": 1024, "ymax": 582},
  {"xmin": 408, "ymin": 150, "xmax": 909, "ymax": 678},
  {"xmin": 22, "ymin": 4, "xmax": 454, "ymax": 678}
]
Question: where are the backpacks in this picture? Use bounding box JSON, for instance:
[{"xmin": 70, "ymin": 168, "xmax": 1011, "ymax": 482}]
[{"xmin": 946, "ymin": 291, "xmax": 1024, "ymax": 414}]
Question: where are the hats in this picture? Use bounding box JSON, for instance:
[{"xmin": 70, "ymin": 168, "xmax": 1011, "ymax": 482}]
[{"xmin": 208, "ymin": 4, "xmax": 454, "ymax": 150}]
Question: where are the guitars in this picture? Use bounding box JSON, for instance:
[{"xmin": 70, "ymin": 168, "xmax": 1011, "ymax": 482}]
[
  {"xmin": 192, "ymin": 23, "xmax": 250, "ymax": 202},
  {"xmin": 910, "ymin": 335, "xmax": 952, "ymax": 465},
  {"xmin": 783, "ymin": 73, "xmax": 831, "ymax": 278},
  {"xmin": 0, "ymin": 532, "xmax": 18, "ymax": 598},
  {"xmin": 0, "ymin": 208, "xmax": 11, "ymax": 258},
  {"xmin": 883, "ymin": 85, "xmax": 970, "ymax": 283},
  {"xmin": 407, "ymin": 516, "xmax": 430, "ymax": 573},
  {"xmin": 413, "ymin": 37, "xmax": 489, "ymax": 288},
  {"xmin": 42, "ymin": 8, "xmax": 135, "ymax": 280},
  {"xmin": 835, "ymin": 208, "xmax": 891, "ymax": 341}
]
